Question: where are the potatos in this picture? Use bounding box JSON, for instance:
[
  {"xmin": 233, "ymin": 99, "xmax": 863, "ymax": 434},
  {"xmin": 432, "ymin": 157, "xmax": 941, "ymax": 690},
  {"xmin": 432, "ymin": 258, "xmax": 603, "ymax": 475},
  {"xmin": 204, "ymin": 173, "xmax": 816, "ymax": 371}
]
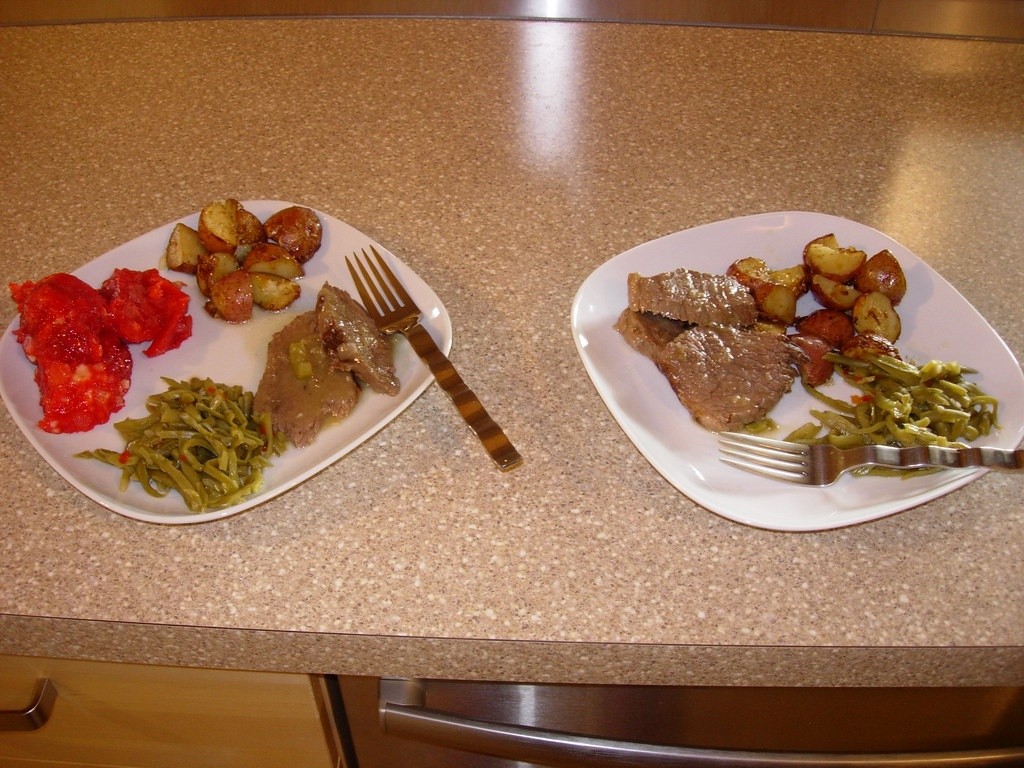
[
  {"xmin": 165, "ymin": 197, "xmax": 324, "ymax": 323},
  {"xmin": 728, "ymin": 233, "xmax": 908, "ymax": 386}
]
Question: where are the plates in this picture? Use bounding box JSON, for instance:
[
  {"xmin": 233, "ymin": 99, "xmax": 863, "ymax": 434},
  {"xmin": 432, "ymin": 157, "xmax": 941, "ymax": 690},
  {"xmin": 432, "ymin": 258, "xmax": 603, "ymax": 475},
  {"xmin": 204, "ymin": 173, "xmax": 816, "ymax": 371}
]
[
  {"xmin": 571, "ymin": 210, "xmax": 1024, "ymax": 531},
  {"xmin": 0, "ymin": 198, "xmax": 453, "ymax": 525}
]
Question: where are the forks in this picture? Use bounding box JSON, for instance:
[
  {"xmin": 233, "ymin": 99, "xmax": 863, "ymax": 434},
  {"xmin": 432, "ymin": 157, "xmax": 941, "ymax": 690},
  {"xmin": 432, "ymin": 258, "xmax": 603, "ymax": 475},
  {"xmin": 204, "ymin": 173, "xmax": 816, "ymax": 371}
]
[
  {"xmin": 343, "ymin": 243, "xmax": 524, "ymax": 473},
  {"xmin": 716, "ymin": 429, "xmax": 1023, "ymax": 488}
]
